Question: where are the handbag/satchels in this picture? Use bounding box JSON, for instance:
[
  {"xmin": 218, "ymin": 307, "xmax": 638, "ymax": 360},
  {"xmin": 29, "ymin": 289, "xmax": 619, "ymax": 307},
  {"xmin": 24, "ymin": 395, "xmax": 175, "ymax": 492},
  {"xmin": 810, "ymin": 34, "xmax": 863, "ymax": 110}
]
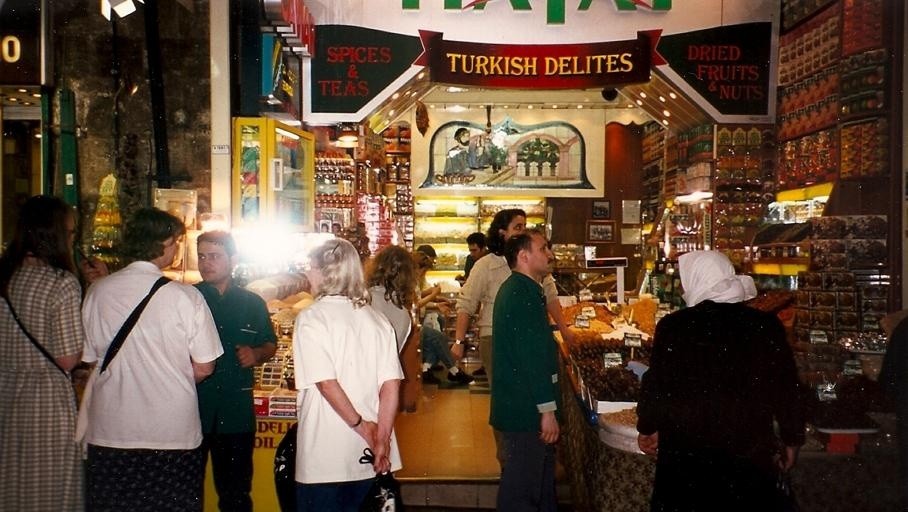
[
  {"xmin": 359, "ymin": 447, "xmax": 407, "ymax": 512},
  {"xmin": 71, "ymin": 366, "xmax": 95, "ymax": 412}
]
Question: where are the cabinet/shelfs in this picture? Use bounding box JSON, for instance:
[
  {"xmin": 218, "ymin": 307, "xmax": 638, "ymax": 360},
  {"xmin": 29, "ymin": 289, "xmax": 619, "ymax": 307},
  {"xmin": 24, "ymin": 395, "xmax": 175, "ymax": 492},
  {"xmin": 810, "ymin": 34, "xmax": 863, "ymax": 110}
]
[
  {"xmin": 421, "ymin": 293, "xmax": 481, "ymax": 364},
  {"xmin": 410, "ymin": 191, "xmax": 546, "ymax": 291},
  {"xmin": 233, "ymin": 117, "xmax": 315, "ymax": 239},
  {"xmin": 311, "ymin": 155, "xmax": 413, "ymax": 255},
  {"xmin": 636, "ymin": 114, "xmax": 715, "ymax": 310}
]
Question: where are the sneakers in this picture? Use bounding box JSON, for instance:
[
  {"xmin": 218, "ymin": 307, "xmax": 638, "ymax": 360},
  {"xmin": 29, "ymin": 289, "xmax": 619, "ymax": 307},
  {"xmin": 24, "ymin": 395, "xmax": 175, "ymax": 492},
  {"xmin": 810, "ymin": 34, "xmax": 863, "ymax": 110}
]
[
  {"xmin": 447, "ymin": 367, "xmax": 476, "ymax": 383},
  {"xmin": 422, "ymin": 368, "xmax": 444, "ymax": 384},
  {"xmin": 472, "ymin": 366, "xmax": 487, "ymax": 375}
]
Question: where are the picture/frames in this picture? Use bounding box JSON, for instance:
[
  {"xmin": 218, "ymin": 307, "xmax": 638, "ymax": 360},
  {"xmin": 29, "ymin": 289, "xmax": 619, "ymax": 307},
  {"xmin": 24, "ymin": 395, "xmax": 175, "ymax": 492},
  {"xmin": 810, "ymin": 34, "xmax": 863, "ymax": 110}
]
[
  {"xmin": 590, "ymin": 198, "xmax": 611, "ymax": 220},
  {"xmin": 584, "ymin": 218, "xmax": 618, "ymax": 243}
]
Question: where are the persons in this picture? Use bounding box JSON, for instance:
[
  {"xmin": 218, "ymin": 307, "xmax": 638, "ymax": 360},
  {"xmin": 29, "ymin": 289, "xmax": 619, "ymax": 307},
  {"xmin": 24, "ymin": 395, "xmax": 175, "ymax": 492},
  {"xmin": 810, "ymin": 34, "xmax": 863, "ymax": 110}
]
[
  {"xmin": 487, "ymin": 229, "xmax": 564, "ymax": 512},
  {"xmin": 73, "ymin": 206, "xmax": 226, "ymax": 512},
  {"xmin": 358, "ymin": 243, "xmax": 418, "ymax": 512},
  {"xmin": 633, "ymin": 251, "xmax": 808, "ymax": 512},
  {"xmin": 875, "ymin": 314, "xmax": 908, "ymax": 511},
  {"xmin": 0, "ymin": 193, "xmax": 87, "ymax": 512},
  {"xmin": 83, "ymin": 228, "xmax": 278, "ymax": 512},
  {"xmin": 412, "ymin": 209, "xmax": 580, "ymax": 394},
  {"xmin": 292, "ymin": 238, "xmax": 406, "ymax": 512},
  {"xmin": 444, "ymin": 128, "xmax": 473, "ymax": 175}
]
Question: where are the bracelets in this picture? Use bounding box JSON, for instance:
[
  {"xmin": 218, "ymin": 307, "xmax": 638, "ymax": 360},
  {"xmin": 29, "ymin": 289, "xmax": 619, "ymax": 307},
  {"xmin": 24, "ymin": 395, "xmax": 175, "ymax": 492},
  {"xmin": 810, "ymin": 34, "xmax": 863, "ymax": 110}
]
[{"xmin": 350, "ymin": 416, "xmax": 362, "ymax": 428}]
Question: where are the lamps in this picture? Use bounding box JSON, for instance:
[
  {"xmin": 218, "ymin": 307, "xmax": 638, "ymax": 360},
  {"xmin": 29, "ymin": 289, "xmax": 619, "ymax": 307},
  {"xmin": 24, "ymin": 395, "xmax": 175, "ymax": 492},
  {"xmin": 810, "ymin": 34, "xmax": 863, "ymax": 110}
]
[{"xmin": 333, "ymin": 121, "xmax": 359, "ymax": 145}]
